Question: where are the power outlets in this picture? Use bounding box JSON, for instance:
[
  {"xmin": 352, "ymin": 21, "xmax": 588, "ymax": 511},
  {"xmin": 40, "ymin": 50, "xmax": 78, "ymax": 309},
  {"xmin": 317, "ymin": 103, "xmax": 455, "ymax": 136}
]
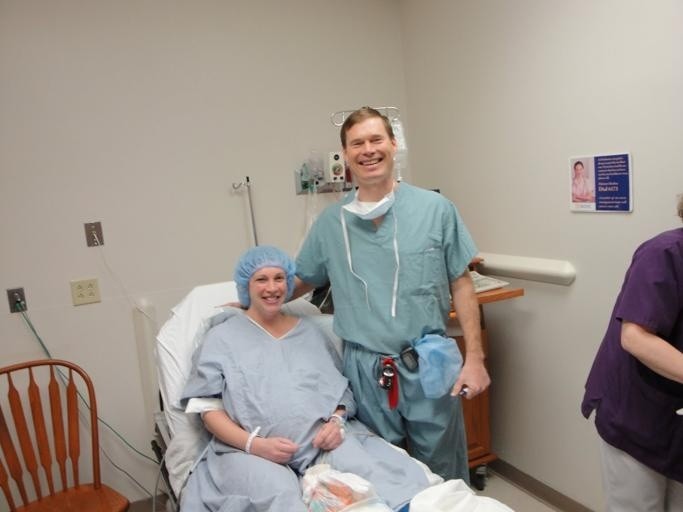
[
  {"xmin": 85, "ymin": 223, "xmax": 104, "ymax": 247},
  {"xmin": 70, "ymin": 279, "xmax": 101, "ymax": 306},
  {"xmin": 7, "ymin": 287, "xmax": 28, "ymax": 314}
]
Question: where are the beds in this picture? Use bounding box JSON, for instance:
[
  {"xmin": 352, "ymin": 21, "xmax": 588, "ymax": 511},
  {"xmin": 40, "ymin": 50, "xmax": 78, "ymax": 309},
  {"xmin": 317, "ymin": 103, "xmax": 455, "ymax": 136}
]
[{"xmin": 140, "ymin": 281, "xmax": 514, "ymax": 512}]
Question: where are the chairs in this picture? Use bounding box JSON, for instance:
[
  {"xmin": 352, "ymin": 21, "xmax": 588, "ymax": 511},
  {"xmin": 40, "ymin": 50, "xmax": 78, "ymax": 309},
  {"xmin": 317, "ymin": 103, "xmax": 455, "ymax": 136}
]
[{"xmin": 1, "ymin": 359, "xmax": 129, "ymax": 512}]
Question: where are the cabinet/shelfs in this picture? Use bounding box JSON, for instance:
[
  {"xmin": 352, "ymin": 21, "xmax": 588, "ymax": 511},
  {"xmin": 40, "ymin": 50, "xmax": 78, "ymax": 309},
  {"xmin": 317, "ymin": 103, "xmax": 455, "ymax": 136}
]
[{"xmin": 446, "ymin": 283, "xmax": 524, "ymax": 490}]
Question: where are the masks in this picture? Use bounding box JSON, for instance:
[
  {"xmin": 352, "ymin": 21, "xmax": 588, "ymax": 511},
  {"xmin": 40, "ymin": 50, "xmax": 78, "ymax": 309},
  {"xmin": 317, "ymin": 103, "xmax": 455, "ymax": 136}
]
[{"xmin": 342, "ymin": 194, "xmax": 394, "ymax": 220}]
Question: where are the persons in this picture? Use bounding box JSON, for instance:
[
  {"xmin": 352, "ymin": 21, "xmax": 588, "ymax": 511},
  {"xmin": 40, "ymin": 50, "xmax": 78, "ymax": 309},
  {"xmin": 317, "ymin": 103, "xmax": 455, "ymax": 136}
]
[
  {"xmin": 178, "ymin": 244, "xmax": 430, "ymax": 512},
  {"xmin": 581, "ymin": 190, "xmax": 682, "ymax": 511},
  {"xmin": 570, "ymin": 160, "xmax": 594, "ymax": 203},
  {"xmin": 210, "ymin": 105, "xmax": 493, "ymax": 493}
]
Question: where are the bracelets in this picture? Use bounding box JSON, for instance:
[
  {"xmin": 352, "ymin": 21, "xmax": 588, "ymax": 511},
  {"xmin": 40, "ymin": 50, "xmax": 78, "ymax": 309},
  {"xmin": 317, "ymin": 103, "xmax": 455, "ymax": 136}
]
[{"xmin": 243, "ymin": 424, "xmax": 266, "ymax": 455}]
